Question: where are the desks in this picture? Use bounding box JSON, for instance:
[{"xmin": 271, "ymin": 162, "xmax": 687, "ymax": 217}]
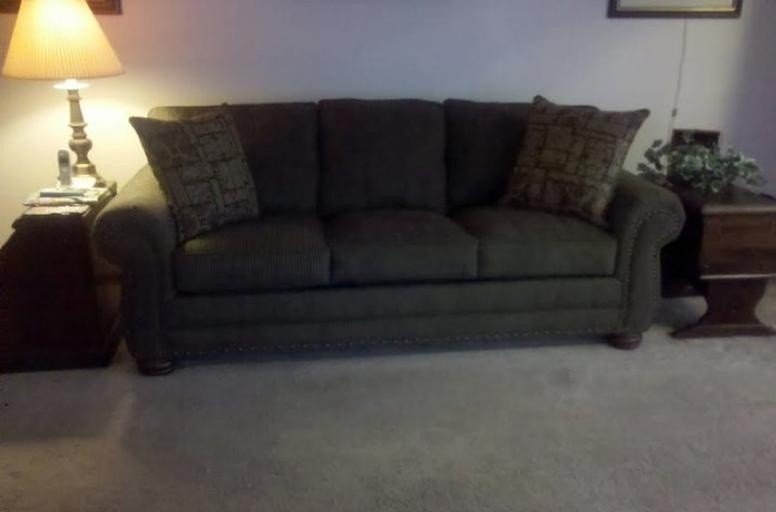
[
  {"xmin": 662, "ymin": 182, "xmax": 775, "ymax": 339},
  {"xmin": 2, "ymin": 182, "xmax": 125, "ymax": 376}
]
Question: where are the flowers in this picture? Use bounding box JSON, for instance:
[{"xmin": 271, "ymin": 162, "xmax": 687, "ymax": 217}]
[{"xmin": 636, "ymin": 131, "xmax": 768, "ymax": 194}]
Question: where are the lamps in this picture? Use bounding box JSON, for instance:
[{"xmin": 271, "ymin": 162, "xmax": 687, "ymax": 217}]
[{"xmin": 1, "ymin": 0, "xmax": 125, "ymax": 188}]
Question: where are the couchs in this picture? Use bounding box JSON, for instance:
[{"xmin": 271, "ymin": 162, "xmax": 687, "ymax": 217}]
[{"xmin": 90, "ymin": 97, "xmax": 685, "ymax": 378}]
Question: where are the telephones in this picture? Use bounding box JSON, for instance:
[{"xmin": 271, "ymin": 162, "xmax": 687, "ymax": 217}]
[{"xmin": 58, "ymin": 149, "xmax": 72, "ymax": 186}]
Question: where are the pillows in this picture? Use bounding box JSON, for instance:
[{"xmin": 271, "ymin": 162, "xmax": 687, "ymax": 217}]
[
  {"xmin": 496, "ymin": 94, "xmax": 650, "ymax": 227},
  {"xmin": 128, "ymin": 101, "xmax": 261, "ymax": 241}
]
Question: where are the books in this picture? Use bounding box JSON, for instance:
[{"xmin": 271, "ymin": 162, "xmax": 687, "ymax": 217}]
[{"xmin": 23, "ymin": 187, "xmax": 111, "ymax": 206}]
